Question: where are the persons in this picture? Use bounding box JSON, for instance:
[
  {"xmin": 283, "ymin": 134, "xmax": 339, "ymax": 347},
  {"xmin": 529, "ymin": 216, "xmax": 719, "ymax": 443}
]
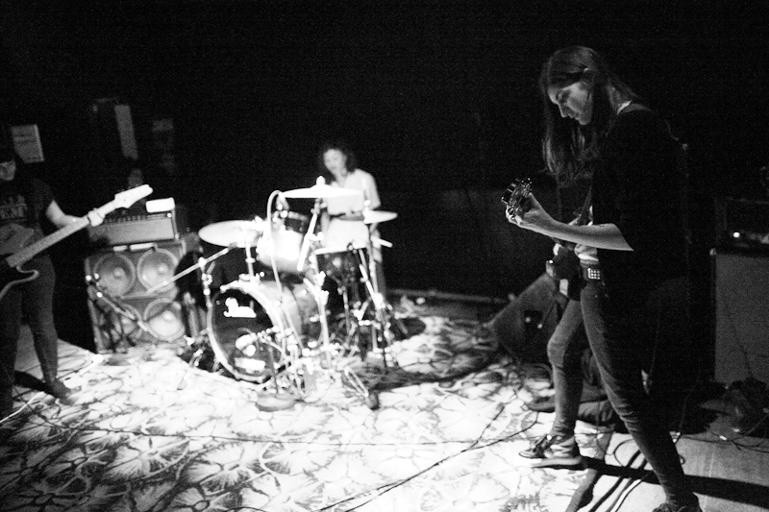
[
  {"xmin": 113, "ymin": 169, "xmax": 152, "ymax": 215},
  {"xmin": 312, "ymin": 139, "xmax": 386, "ymax": 294},
  {"xmin": 503, "ymin": 43, "xmax": 707, "ymax": 511},
  {"xmin": 0, "ymin": 147, "xmax": 105, "ymax": 416}
]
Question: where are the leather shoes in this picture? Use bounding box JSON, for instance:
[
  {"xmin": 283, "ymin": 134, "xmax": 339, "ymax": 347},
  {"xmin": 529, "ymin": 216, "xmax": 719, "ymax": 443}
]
[{"xmin": 45, "ymin": 379, "xmax": 72, "ymax": 398}]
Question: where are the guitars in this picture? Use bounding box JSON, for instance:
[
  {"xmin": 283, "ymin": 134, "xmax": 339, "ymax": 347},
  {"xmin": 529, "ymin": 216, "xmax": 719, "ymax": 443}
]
[
  {"xmin": 496, "ymin": 176, "xmax": 586, "ymax": 301},
  {"xmin": 0, "ymin": 184, "xmax": 154, "ymax": 299}
]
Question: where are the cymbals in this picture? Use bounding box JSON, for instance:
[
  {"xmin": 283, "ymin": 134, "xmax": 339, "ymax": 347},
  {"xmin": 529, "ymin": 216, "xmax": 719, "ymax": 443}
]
[
  {"xmin": 280, "ymin": 184, "xmax": 363, "ymax": 199},
  {"xmin": 339, "ymin": 209, "xmax": 397, "ymax": 224}
]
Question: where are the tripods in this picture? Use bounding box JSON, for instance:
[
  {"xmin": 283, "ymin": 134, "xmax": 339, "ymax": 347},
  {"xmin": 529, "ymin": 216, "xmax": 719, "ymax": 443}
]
[{"xmin": 362, "ymin": 225, "xmax": 422, "ymax": 392}]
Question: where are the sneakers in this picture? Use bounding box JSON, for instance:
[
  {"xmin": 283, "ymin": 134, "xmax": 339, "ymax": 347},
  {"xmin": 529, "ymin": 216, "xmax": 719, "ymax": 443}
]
[
  {"xmin": 518, "ymin": 433, "xmax": 582, "ymax": 468},
  {"xmin": 652, "ymin": 497, "xmax": 703, "ymax": 512}
]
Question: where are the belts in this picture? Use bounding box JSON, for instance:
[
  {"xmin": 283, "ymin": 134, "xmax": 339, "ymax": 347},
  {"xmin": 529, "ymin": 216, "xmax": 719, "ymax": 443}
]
[{"xmin": 579, "ymin": 266, "xmax": 602, "ymax": 282}]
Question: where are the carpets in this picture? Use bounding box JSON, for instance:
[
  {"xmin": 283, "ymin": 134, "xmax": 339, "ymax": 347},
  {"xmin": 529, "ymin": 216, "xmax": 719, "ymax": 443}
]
[{"xmin": 0, "ymin": 315, "xmax": 615, "ymax": 512}]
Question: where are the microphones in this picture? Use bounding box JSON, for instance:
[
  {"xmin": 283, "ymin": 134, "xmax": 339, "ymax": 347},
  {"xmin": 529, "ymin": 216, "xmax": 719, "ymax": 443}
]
[{"xmin": 279, "ymin": 192, "xmax": 289, "ymax": 210}]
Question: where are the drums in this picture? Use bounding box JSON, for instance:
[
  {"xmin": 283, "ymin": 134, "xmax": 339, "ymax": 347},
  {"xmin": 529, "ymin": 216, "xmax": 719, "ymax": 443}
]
[
  {"xmin": 313, "ymin": 247, "xmax": 376, "ymax": 320},
  {"xmin": 207, "ymin": 275, "xmax": 323, "ymax": 383},
  {"xmin": 266, "ymin": 210, "xmax": 317, "ymax": 235},
  {"xmin": 197, "ymin": 219, "xmax": 264, "ymax": 289}
]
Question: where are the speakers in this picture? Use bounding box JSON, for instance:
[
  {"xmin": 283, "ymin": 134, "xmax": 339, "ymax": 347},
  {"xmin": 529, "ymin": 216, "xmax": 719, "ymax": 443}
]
[
  {"xmin": 84, "ymin": 233, "xmax": 202, "ymax": 354},
  {"xmin": 708, "ymin": 240, "xmax": 769, "ymax": 387}
]
